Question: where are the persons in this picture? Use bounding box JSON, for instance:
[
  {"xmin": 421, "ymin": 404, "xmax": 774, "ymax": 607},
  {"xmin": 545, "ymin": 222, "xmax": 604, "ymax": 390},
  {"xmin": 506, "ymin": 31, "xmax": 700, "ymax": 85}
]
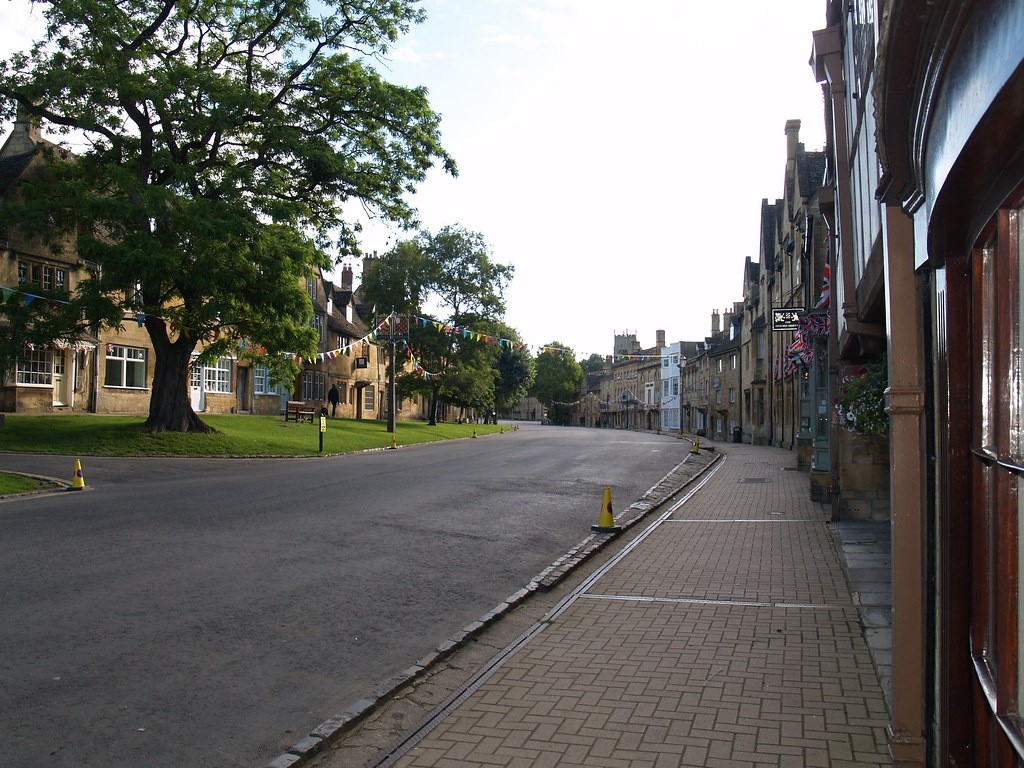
[{"xmin": 328, "ymin": 384, "xmax": 339, "ymax": 417}]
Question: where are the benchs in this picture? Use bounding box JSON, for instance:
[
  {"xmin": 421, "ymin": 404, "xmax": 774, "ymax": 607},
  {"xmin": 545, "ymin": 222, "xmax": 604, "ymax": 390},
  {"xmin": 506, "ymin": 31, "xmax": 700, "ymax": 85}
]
[{"xmin": 285, "ymin": 400, "xmax": 316, "ymax": 424}]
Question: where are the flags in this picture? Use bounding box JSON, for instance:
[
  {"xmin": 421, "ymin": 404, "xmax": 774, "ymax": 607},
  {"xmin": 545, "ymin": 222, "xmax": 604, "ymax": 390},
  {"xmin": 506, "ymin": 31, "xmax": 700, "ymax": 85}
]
[{"xmin": 773, "ymin": 234, "xmax": 830, "ymax": 381}]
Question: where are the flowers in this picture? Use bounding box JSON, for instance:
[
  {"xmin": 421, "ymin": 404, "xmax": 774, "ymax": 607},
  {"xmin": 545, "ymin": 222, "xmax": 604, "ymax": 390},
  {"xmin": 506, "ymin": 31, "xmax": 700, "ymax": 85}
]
[{"xmin": 832, "ymin": 351, "xmax": 887, "ymax": 441}]
